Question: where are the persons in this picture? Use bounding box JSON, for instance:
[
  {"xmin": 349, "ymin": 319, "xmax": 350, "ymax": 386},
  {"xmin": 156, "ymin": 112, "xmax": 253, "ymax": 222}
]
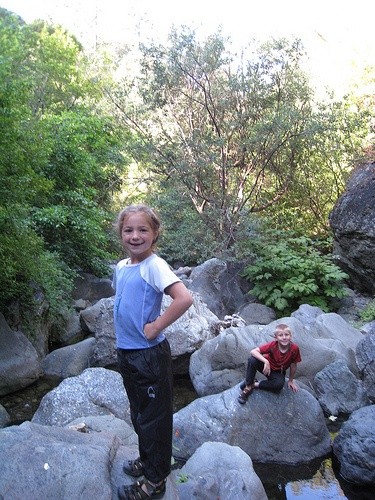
[
  {"xmin": 110, "ymin": 205, "xmax": 193, "ymax": 500},
  {"xmin": 237, "ymin": 324, "xmax": 302, "ymax": 404}
]
